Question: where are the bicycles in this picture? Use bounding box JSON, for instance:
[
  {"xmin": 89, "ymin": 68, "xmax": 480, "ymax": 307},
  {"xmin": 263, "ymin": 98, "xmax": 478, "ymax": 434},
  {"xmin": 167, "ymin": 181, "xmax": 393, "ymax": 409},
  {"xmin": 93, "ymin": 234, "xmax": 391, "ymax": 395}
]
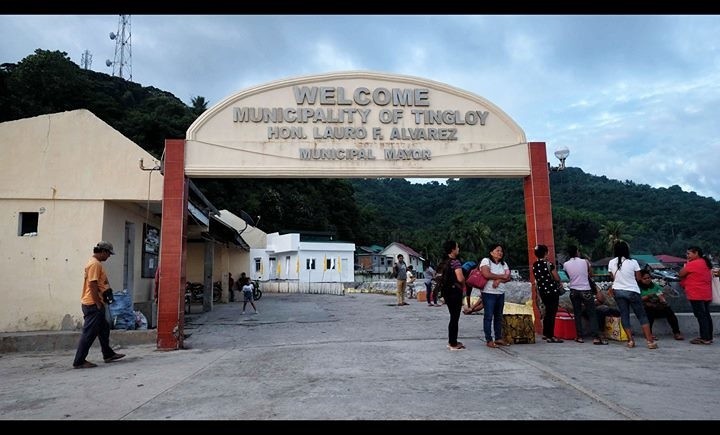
[{"xmin": 252, "ymin": 276, "xmax": 262, "ymax": 300}]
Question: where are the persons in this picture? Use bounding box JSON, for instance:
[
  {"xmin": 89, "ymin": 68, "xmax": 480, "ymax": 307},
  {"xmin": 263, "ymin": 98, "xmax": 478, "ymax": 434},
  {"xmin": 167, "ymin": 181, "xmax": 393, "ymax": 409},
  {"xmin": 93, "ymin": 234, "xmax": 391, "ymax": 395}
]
[
  {"xmin": 406, "ymin": 264, "xmax": 417, "ymax": 299},
  {"xmin": 608, "ymin": 240, "xmax": 658, "ymax": 349},
  {"xmin": 73, "ymin": 241, "xmax": 126, "ymax": 369},
  {"xmin": 236, "ymin": 272, "xmax": 248, "ymax": 292},
  {"xmin": 439, "ymin": 240, "xmax": 467, "ymax": 351},
  {"xmin": 393, "ymin": 254, "xmax": 410, "ymax": 306},
  {"xmin": 478, "ymin": 243, "xmax": 510, "ymax": 347},
  {"xmin": 594, "ymin": 245, "xmax": 714, "ymax": 344},
  {"xmin": 240, "ymin": 277, "xmax": 258, "ymax": 315},
  {"xmin": 229, "ymin": 272, "xmax": 236, "ymax": 302},
  {"xmin": 533, "ymin": 244, "xmax": 564, "ymax": 343},
  {"xmin": 561, "ymin": 244, "xmax": 607, "ymax": 345},
  {"xmin": 423, "ymin": 259, "xmax": 441, "ymax": 307},
  {"xmin": 462, "ymin": 261, "xmax": 478, "ymax": 308},
  {"xmin": 462, "ymin": 297, "xmax": 484, "ymax": 315}
]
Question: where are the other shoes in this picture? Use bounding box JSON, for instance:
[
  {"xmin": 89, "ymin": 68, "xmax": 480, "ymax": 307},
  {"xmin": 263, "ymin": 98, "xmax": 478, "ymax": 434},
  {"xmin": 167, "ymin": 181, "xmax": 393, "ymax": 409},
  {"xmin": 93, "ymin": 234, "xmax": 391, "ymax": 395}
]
[
  {"xmin": 240, "ymin": 312, "xmax": 245, "ymax": 315},
  {"xmin": 255, "ymin": 311, "xmax": 258, "ymax": 314},
  {"xmin": 184, "ymin": 333, "xmax": 192, "ymax": 339},
  {"xmin": 103, "ymin": 353, "xmax": 127, "ymax": 363},
  {"xmin": 73, "ymin": 360, "xmax": 98, "ymax": 368},
  {"xmin": 397, "ymin": 296, "xmax": 442, "ymax": 307}
]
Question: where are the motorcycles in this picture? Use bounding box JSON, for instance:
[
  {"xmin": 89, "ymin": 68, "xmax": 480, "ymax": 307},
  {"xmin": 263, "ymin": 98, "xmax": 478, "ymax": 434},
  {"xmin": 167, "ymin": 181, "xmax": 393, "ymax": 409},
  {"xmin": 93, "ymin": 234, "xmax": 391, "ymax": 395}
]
[{"xmin": 185, "ymin": 280, "xmax": 222, "ymax": 305}]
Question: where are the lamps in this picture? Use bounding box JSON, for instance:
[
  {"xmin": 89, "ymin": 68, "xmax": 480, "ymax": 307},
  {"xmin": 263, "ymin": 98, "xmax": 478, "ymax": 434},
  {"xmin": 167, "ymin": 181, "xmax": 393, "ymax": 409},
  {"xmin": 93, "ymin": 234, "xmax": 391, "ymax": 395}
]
[{"xmin": 547, "ymin": 149, "xmax": 569, "ymax": 175}]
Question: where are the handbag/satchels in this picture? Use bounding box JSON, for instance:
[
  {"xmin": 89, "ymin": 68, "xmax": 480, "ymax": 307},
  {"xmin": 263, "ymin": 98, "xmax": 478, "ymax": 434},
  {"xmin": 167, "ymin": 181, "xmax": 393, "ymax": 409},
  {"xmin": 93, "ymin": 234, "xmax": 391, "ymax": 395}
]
[
  {"xmin": 502, "ymin": 314, "xmax": 536, "ymax": 345},
  {"xmin": 446, "ymin": 334, "xmax": 715, "ymax": 351},
  {"xmin": 102, "ymin": 288, "xmax": 115, "ymax": 305},
  {"xmin": 604, "ymin": 314, "xmax": 629, "ymax": 341},
  {"xmin": 606, "ymin": 286, "xmax": 615, "ymax": 298},
  {"xmin": 547, "ymin": 260, "xmax": 566, "ymax": 296},
  {"xmin": 465, "ymin": 261, "xmax": 490, "ymax": 290},
  {"xmin": 588, "ymin": 272, "xmax": 597, "ymax": 295}
]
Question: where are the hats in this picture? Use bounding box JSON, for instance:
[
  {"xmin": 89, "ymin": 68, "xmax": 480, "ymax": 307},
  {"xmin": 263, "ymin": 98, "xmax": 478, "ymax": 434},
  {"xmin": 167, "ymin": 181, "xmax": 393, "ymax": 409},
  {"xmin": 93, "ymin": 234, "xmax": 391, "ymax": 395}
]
[{"xmin": 94, "ymin": 241, "xmax": 116, "ymax": 256}]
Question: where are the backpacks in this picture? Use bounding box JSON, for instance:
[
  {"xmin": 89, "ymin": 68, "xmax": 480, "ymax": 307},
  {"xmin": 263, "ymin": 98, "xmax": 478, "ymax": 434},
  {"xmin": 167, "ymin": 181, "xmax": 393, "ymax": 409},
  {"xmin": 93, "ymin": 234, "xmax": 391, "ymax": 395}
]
[{"xmin": 434, "ymin": 257, "xmax": 461, "ymax": 287}]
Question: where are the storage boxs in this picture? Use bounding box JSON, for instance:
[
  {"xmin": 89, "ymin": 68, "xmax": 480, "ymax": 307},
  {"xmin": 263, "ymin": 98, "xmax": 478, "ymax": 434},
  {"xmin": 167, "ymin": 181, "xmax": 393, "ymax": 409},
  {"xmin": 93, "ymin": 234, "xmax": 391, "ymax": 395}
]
[
  {"xmin": 501, "ymin": 312, "xmax": 536, "ymax": 345},
  {"xmin": 553, "ymin": 311, "xmax": 577, "ymax": 341}
]
